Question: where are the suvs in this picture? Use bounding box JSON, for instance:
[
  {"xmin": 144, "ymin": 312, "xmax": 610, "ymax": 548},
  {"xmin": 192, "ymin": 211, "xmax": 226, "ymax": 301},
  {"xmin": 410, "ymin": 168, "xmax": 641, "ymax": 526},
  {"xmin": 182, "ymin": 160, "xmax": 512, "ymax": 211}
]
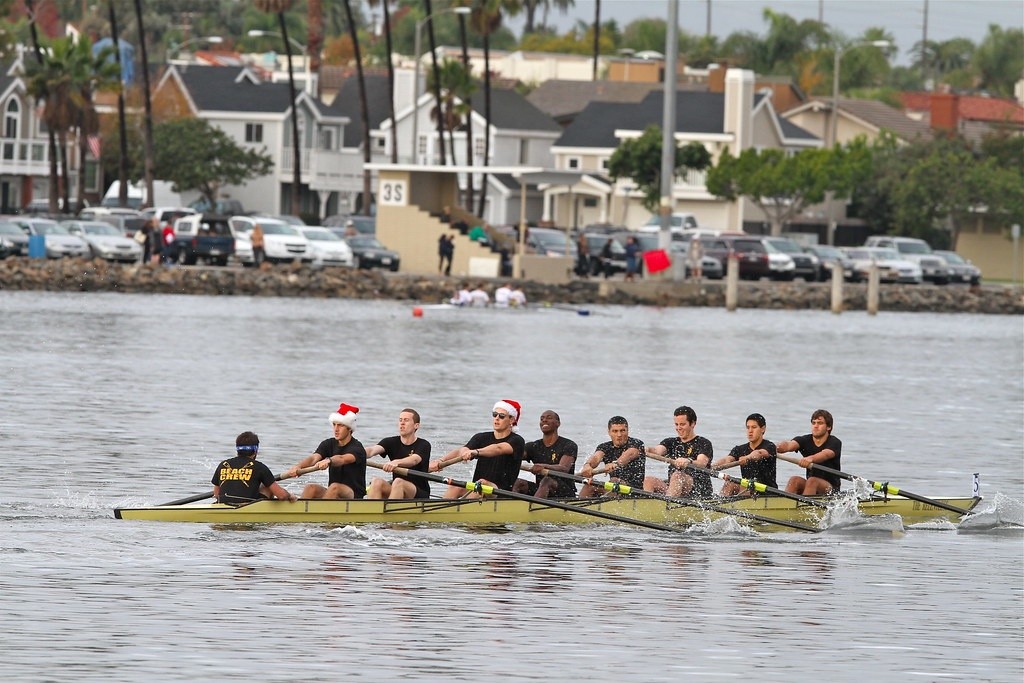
[{"xmin": 864, "ymin": 235, "xmax": 949, "ymax": 286}]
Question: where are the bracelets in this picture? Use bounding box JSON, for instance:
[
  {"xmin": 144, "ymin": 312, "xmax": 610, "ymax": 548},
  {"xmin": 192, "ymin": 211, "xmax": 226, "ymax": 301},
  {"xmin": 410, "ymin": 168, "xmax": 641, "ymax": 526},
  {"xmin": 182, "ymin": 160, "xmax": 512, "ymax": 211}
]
[
  {"xmin": 476, "ymin": 449, "xmax": 480, "ymax": 457},
  {"xmin": 612, "ymin": 461, "xmax": 620, "ymax": 466},
  {"xmin": 688, "ymin": 458, "xmax": 693, "ymax": 464}
]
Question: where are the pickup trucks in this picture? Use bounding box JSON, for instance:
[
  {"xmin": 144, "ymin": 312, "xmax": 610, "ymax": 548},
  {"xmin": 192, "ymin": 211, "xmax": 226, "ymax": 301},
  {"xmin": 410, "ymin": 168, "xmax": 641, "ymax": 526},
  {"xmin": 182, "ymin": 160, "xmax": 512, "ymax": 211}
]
[{"xmin": 146, "ymin": 207, "xmax": 236, "ymax": 267}]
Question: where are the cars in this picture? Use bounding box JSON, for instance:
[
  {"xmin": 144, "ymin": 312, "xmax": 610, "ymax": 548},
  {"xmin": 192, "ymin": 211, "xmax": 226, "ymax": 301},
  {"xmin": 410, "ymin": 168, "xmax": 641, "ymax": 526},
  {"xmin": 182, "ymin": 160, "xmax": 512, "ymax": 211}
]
[
  {"xmin": 0, "ymin": 205, "xmax": 152, "ymax": 263},
  {"xmin": 226, "ymin": 215, "xmax": 402, "ymax": 273},
  {"xmin": 936, "ymin": 248, "xmax": 982, "ymax": 287},
  {"xmin": 808, "ymin": 244, "xmax": 923, "ymax": 286}
]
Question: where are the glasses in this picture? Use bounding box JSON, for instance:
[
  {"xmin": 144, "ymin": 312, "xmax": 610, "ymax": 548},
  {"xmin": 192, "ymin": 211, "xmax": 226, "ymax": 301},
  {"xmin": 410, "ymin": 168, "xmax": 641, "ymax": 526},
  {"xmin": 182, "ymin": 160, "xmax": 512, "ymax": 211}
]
[{"xmin": 492, "ymin": 412, "xmax": 510, "ymax": 420}]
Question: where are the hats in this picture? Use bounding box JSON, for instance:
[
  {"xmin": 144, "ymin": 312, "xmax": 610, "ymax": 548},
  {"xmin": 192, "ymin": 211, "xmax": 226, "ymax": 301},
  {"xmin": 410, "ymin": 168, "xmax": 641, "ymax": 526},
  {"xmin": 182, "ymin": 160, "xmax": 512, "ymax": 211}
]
[
  {"xmin": 329, "ymin": 403, "xmax": 359, "ymax": 432},
  {"xmin": 492, "ymin": 399, "xmax": 521, "ymax": 422},
  {"xmin": 346, "ymin": 220, "xmax": 354, "ymax": 226}
]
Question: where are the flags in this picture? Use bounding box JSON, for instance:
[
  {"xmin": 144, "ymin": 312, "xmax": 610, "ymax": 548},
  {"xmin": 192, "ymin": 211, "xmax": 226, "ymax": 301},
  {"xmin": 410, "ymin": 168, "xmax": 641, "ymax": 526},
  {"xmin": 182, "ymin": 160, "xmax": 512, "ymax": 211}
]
[{"xmin": 88, "ymin": 132, "xmax": 103, "ymax": 158}]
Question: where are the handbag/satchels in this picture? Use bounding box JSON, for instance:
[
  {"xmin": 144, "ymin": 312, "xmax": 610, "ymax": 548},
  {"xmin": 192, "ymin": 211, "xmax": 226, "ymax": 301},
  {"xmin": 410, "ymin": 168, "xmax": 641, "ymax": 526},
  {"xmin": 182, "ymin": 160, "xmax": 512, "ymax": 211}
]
[
  {"xmin": 134, "ymin": 229, "xmax": 146, "ymax": 244},
  {"xmin": 166, "ymin": 233, "xmax": 174, "ymax": 244}
]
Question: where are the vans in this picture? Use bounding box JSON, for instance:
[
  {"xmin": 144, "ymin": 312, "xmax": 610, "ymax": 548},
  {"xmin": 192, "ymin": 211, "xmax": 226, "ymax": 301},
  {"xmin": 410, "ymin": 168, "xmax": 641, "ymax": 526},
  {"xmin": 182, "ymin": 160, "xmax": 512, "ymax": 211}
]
[
  {"xmin": 488, "ymin": 213, "xmax": 796, "ymax": 282},
  {"xmin": 98, "ymin": 177, "xmax": 180, "ymax": 213}
]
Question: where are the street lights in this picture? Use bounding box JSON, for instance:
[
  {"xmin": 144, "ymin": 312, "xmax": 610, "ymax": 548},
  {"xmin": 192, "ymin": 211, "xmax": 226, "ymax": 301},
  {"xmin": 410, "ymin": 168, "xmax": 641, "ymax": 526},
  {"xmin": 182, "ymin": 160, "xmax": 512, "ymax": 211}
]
[
  {"xmin": 412, "ymin": 6, "xmax": 474, "ymax": 164},
  {"xmin": 828, "ymin": 40, "xmax": 892, "ymax": 246},
  {"xmin": 246, "ymin": 29, "xmax": 309, "ymax": 101}
]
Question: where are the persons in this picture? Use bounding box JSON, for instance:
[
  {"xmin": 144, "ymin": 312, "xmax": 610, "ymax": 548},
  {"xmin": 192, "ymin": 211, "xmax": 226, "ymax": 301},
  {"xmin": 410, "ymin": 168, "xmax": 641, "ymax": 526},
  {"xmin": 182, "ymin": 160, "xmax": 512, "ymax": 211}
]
[
  {"xmin": 579, "ymin": 416, "xmax": 646, "ymax": 498},
  {"xmin": 450, "ymin": 281, "xmax": 527, "ymax": 307},
  {"xmin": 775, "ymin": 410, "xmax": 842, "ymax": 495},
  {"xmin": 711, "ymin": 413, "xmax": 778, "ymax": 497},
  {"xmin": 643, "ymin": 406, "xmax": 713, "ymax": 498},
  {"xmin": 685, "ymin": 233, "xmax": 704, "ymax": 280},
  {"xmin": 211, "ymin": 432, "xmax": 290, "ymax": 503},
  {"xmin": 364, "ymin": 408, "xmax": 431, "ymax": 499},
  {"xmin": 429, "ymin": 399, "xmax": 524, "ymax": 499},
  {"xmin": 250, "ymin": 223, "xmax": 266, "ymax": 263},
  {"xmin": 141, "ymin": 216, "xmax": 176, "ymax": 263},
  {"xmin": 623, "ymin": 235, "xmax": 642, "ymax": 281},
  {"xmin": 442, "ymin": 204, "xmax": 489, "ymax": 240},
  {"xmin": 343, "ymin": 220, "xmax": 358, "ymax": 236},
  {"xmin": 289, "ymin": 402, "xmax": 366, "ymax": 498},
  {"xmin": 512, "ymin": 410, "xmax": 578, "ymax": 499},
  {"xmin": 577, "ymin": 234, "xmax": 613, "ymax": 278},
  {"xmin": 439, "ymin": 234, "xmax": 455, "ymax": 274}
]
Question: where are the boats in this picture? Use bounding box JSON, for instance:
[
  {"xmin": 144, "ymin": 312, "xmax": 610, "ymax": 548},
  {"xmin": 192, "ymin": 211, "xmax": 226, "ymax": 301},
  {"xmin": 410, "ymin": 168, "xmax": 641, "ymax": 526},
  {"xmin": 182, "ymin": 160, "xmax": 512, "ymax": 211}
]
[
  {"xmin": 412, "ymin": 297, "xmax": 548, "ymax": 323},
  {"xmin": 111, "ymin": 496, "xmax": 983, "ymax": 531}
]
